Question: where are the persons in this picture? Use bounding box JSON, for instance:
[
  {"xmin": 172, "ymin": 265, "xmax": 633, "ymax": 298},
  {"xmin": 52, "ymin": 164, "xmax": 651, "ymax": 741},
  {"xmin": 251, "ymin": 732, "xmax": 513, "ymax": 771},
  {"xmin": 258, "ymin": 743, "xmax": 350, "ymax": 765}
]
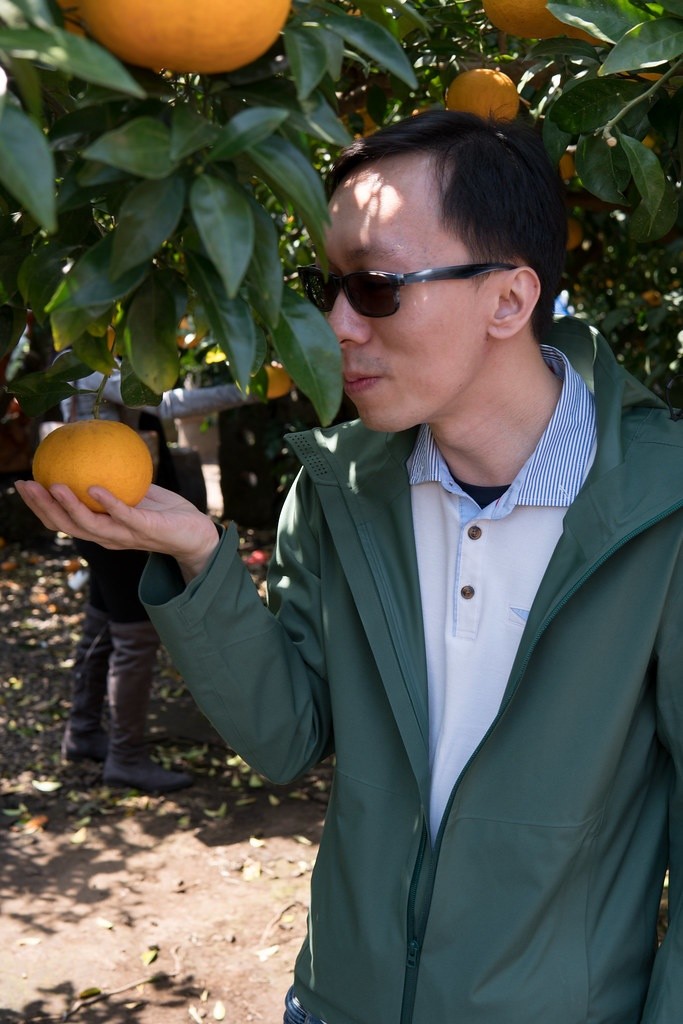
[
  {"xmin": 14, "ymin": 109, "xmax": 683, "ymax": 1024},
  {"xmin": 60, "ymin": 334, "xmax": 296, "ymax": 789}
]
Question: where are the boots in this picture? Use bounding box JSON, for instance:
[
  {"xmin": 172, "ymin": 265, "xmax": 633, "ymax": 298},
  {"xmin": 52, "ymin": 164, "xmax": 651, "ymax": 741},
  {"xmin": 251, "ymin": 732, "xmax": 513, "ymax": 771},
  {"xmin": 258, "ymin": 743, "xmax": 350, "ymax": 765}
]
[
  {"xmin": 98, "ymin": 618, "xmax": 198, "ymax": 797},
  {"xmin": 61, "ymin": 599, "xmax": 106, "ymax": 763}
]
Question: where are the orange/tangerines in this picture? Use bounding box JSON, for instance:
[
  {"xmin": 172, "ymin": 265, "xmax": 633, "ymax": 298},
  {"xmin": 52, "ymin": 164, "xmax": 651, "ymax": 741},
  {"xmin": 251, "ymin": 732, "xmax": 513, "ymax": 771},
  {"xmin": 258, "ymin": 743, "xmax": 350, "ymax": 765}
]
[
  {"xmin": 50, "ymin": 0, "xmax": 668, "ymax": 397},
  {"xmin": 33, "ymin": 418, "xmax": 153, "ymax": 514}
]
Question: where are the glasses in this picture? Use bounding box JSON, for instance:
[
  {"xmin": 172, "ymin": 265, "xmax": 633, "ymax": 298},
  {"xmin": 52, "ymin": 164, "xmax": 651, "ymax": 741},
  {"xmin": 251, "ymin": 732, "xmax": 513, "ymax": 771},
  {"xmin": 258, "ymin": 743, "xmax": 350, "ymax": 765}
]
[{"xmin": 297, "ymin": 262, "xmax": 519, "ymax": 318}]
